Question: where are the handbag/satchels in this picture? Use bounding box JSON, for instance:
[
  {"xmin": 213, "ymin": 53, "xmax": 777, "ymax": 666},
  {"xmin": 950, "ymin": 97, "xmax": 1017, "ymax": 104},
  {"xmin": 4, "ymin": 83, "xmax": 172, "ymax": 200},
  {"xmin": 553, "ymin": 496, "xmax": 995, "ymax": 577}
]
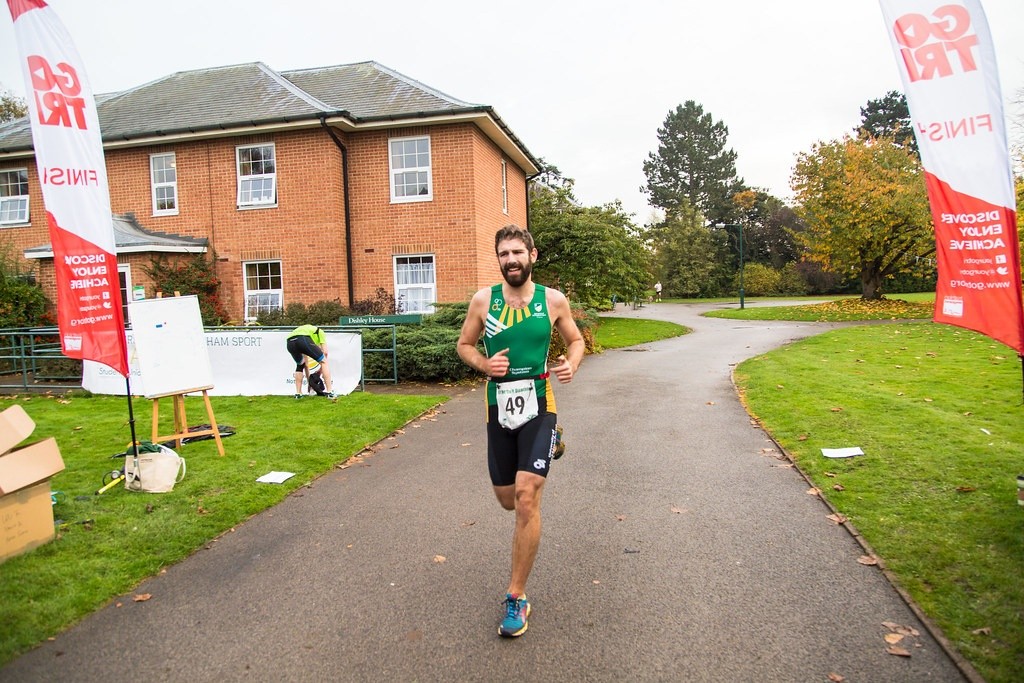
[{"xmin": 124, "ymin": 447, "xmax": 186, "ymax": 494}]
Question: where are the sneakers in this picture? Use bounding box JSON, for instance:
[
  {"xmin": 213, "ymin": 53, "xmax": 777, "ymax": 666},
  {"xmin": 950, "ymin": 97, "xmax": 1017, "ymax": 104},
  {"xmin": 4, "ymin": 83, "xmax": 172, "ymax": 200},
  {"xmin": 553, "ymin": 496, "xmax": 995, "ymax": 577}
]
[
  {"xmin": 553, "ymin": 425, "xmax": 564, "ymax": 461},
  {"xmin": 497, "ymin": 593, "xmax": 530, "ymax": 638}
]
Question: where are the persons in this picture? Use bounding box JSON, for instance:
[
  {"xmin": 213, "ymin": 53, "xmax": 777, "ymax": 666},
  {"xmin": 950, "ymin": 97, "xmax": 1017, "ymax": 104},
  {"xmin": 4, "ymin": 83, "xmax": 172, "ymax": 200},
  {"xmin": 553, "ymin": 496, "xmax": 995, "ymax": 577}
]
[
  {"xmin": 654, "ymin": 280, "xmax": 662, "ymax": 303},
  {"xmin": 457, "ymin": 224, "xmax": 585, "ymax": 641},
  {"xmin": 286, "ymin": 325, "xmax": 339, "ymax": 399}
]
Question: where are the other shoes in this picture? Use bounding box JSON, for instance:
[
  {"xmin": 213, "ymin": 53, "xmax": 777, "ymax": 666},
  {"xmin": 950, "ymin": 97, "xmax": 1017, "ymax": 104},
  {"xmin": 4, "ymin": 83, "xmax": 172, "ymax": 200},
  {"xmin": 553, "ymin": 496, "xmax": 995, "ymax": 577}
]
[
  {"xmin": 294, "ymin": 395, "xmax": 302, "ymax": 399},
  {"xmin": 326, "ymin": 392, "xmax": 336, "ymax": 399}
]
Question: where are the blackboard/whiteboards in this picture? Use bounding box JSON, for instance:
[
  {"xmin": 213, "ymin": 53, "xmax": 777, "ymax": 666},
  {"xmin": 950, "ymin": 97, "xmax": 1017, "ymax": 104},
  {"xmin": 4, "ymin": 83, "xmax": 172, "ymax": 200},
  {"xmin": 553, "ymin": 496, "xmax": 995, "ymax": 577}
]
[{"xmin": 127, "ymin": 294, "xmax": 215, "ymax": 400}]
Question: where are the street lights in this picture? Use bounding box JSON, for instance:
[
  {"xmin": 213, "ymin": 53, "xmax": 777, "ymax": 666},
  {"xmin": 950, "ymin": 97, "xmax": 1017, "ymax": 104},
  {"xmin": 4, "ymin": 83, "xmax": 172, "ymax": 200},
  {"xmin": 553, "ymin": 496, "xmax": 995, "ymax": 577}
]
[{"xmin": 715, "ymin": 222, "xmax": 744, "ymax": 309}]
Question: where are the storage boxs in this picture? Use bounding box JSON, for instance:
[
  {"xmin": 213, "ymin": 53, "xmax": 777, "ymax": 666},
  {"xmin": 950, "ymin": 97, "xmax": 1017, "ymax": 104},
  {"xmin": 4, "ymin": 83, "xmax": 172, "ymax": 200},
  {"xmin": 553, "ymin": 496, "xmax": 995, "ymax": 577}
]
[{"xmin": 0, "ymin": 405, "xmax": 66, "ymax": 561}]
